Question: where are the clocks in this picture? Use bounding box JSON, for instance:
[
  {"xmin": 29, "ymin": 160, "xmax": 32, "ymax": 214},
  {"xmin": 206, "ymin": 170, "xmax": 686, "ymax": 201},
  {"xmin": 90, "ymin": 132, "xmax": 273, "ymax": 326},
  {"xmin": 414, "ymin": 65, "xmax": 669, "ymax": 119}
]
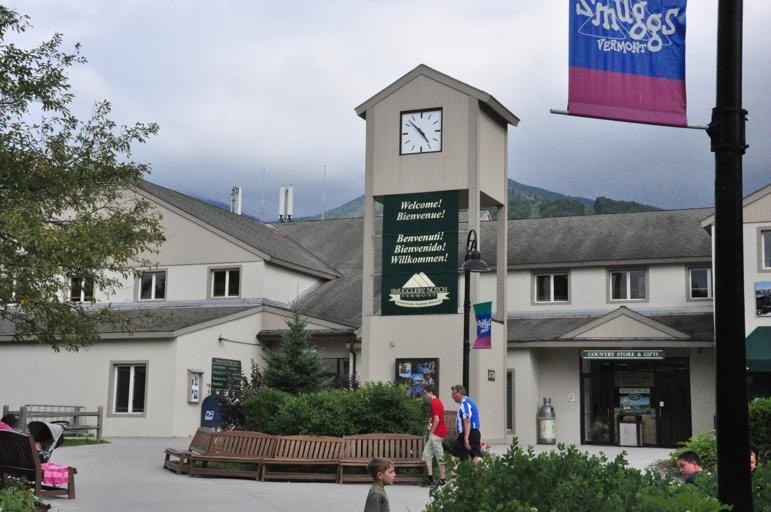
[{"xmin": 399, "ymin": 105, "xmax": 444, "ymax": 156}]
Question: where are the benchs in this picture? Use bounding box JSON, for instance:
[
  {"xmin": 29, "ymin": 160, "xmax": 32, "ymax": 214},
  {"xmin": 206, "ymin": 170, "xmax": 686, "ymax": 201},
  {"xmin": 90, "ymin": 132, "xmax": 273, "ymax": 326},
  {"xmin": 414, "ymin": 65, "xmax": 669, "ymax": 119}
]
[
  {"xmin": 0, "ymin": 430, "xmax": 78, "ymax": 503},
  {"xmin": 161, "ymin": 421, "xmax": 437, "ymax": 486}
]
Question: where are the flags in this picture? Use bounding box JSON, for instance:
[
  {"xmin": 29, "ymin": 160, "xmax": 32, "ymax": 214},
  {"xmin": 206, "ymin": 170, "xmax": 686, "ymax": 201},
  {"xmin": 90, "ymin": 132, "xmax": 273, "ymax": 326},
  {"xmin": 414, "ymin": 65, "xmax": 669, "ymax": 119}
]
[
  {"xmin": 567, "ymin": 0, "xmax": 688, "ymax": 130},
  {"xmin": 473, "ymin": 301, "xmax": 493, "ymax": 349}
]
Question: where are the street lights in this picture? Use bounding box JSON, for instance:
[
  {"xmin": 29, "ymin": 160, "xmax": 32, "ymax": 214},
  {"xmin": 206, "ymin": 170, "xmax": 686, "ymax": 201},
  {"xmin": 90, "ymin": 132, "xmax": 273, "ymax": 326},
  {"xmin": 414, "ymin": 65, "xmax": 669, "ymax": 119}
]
[{"xmin": 461, "ymin": 223, "xmax": 492, "ymax": 400}]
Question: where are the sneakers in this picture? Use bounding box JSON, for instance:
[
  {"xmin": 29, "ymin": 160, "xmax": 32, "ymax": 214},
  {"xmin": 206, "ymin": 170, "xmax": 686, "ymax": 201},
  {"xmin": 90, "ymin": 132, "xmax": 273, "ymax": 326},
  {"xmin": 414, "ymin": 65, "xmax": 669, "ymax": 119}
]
[
  {"xmin": 420, "ymin": 480, "xmax": 435, "ymax": 487},
  {"xmin": 437, "ymin": 479, "xmax": 447, "ymax": 488}
]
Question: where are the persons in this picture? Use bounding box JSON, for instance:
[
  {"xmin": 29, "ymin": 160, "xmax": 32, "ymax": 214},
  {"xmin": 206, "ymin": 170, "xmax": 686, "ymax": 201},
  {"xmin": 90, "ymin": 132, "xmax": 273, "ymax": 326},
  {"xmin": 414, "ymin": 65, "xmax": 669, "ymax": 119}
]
[
  {"xmin": 449, "ymin": 384, "xmax": 489, "ymax": 491},
  {"xmin": 421, "ymin": 384, "xmax": 448, "ymax": 487},
  {"xmin": 365, "ymin": 457, "xmax": 398, "ymax": 512},
  {"xmin": 676, "ymin": 449, "xmax": 709, "ymax": 485},
  {"xmin": 398, "ymin": 361, "xmax": 436, "ymax": 401},
  {"xmin": 0, "ymin": 414, "xmax": 18, "ymax": 433},
  {"xmin": 750, "ymin": 448, "xmax": 763, "ymax": 474}
]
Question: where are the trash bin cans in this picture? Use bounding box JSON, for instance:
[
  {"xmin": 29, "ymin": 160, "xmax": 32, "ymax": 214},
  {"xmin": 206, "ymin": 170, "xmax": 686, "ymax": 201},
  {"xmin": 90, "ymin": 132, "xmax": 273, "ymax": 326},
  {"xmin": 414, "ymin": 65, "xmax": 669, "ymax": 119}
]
[{"xmin": 617, "ymin": 413, "xmax": 643, "ymax": 447}]
